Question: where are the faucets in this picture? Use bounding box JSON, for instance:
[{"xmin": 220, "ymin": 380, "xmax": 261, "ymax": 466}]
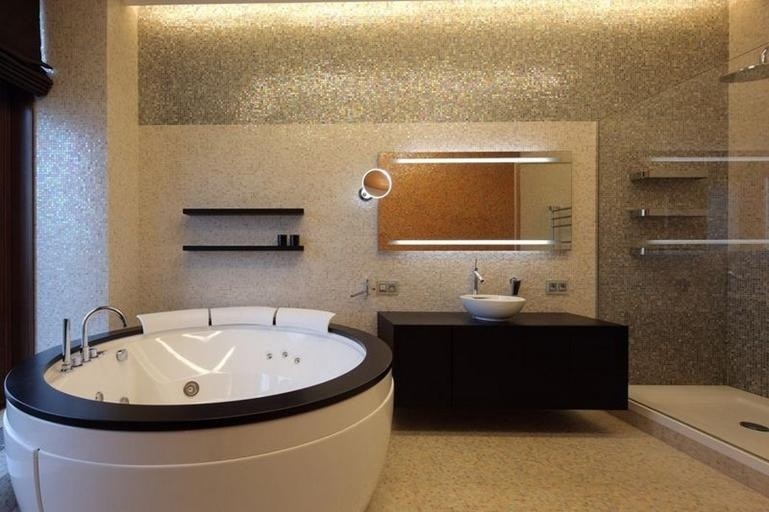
[
  {"xmin": 81, "ymin": 304, "xmax": 128, "ymax": 347},
  {"xmin": 473, "ymin": 258, "xmax": 485, "ymax": 294}
]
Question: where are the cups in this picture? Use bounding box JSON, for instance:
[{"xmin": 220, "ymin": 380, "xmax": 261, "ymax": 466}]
[{"xmin": 277, "ymin": 231, "xmax": 299, "ymax": 248}]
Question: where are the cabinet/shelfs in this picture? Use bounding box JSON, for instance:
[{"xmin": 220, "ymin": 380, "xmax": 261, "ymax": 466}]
[
  {"xmin": 549, "ymin": 205, "xmax": 572, "ymax": 251},
  {"xmin": 182, "ymin": 208, "xmax": 304, "ymax": 251},
  {"xmin": 632, "ymin": 170, "xmax": 710, "ymax": 254}
]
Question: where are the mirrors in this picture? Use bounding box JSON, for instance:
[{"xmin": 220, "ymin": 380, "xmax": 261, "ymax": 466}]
[{"xmin": 377, "ymin": 150, "xmax": 573, "ymax": 251}]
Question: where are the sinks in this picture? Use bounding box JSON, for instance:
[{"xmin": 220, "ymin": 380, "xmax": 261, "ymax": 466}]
[{"xmin": 458, "ymin": 295, "xmax": 525, "ymax": 322}]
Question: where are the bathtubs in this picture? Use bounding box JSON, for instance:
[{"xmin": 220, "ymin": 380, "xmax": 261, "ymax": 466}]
[{"xmin": 43, "ymin": 325, "xmax": 367, "ymax": 406}]
